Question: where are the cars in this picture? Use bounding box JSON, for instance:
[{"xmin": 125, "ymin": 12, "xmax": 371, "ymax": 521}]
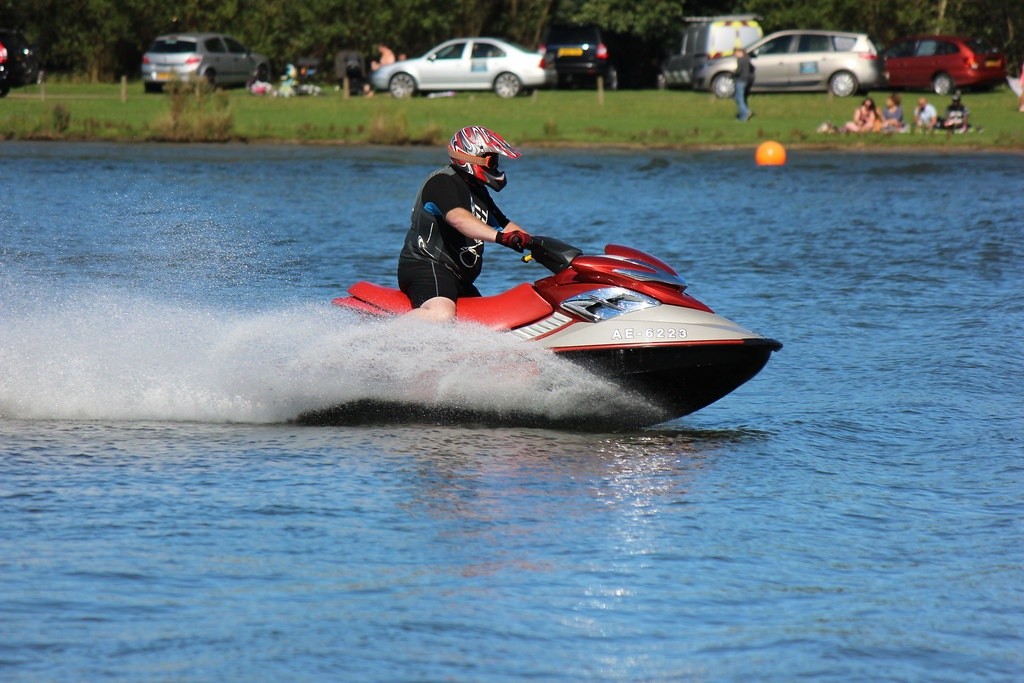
[
  {"xmin": 697, "ymin": 28, "xmax": 885, "ymax": 99},
  {"xmin": 0, "ymin": 32, "xmax": 40, "ymax": 97},
  {"xmin": 877, "ymin": 32, "xmax": 1007, "ymax": 95},
  {"xmin": 370, "ymin": 36, "xmax": 559, "ymax": 99}
]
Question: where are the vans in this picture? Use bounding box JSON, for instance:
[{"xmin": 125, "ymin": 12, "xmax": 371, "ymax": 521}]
[{"xmin": 657, "ymin": 18, "xmax": 763, "ymax": 89}]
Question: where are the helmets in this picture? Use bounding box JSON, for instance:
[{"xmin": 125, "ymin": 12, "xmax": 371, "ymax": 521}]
[{"xmin": 449, "ymin": 125, "xmax": 522, "ymax": 192}]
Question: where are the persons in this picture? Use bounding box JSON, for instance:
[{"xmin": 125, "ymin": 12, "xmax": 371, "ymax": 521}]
[
  {"xmin": 845, "ymin": 92, "xmax": 906, "ymax": 134},
  {"xmin": 364, "ymin": 44, "xmax": 395, "ymax": 96},
  {"xmin": 728, "ymin": 47, "xmax": 755, "ymax": 121},
  {"xmin": 1018, "ymin": 63, "xmax": 1024, "ymax": 112},
  {"xmin": 914, "ymin": 96, "xmax": 937, "ymax": 130},
  {"xmin": 943, "ymin": 95, "xmax": 969, "ymax": 134},
  {"xmin": 397, "ymin": 126, "xmax": 535, "ymax": 323}
]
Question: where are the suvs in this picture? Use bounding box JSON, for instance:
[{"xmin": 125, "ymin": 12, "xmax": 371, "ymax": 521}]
[
  {"xmin": 143, "ymin": 34, "xmax": 273, "ymax": 94},
  {"xmin": 538, "ymin": 21, "xmax": 634, "ymax": 91}
]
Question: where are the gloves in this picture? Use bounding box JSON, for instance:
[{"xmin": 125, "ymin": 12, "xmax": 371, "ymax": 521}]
[{"xmin": 495, "ymin": 230, "xmax": 532, "ymax": 253}]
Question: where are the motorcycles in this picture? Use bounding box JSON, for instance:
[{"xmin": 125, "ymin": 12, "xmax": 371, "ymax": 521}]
[{"xmin": 329, "ymin": 244, "xmax": 786, "ymax": 424}]
